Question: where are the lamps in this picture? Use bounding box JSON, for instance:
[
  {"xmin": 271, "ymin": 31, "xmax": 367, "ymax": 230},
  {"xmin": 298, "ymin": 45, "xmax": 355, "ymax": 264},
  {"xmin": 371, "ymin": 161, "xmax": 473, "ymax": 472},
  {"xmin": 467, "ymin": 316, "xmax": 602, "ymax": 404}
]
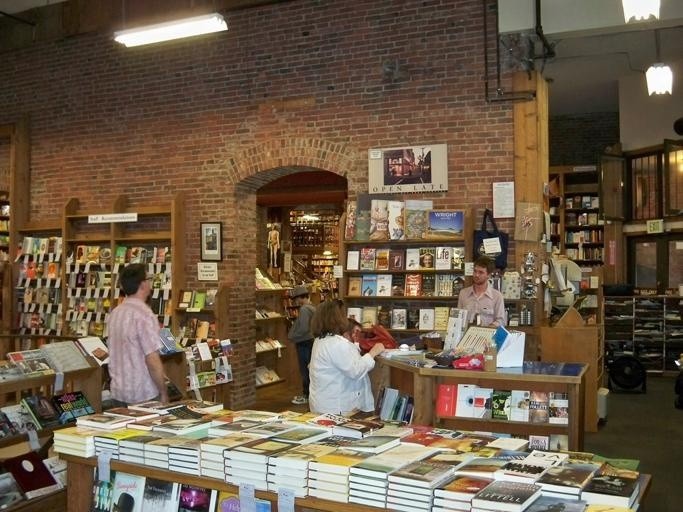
[
  {"xmin": 113, "ymin": 12, "xmax": 229, "ymax": 48},
  {"xmin": 645, "ymin": 29, "xmax": 672, "ymax": 95}
]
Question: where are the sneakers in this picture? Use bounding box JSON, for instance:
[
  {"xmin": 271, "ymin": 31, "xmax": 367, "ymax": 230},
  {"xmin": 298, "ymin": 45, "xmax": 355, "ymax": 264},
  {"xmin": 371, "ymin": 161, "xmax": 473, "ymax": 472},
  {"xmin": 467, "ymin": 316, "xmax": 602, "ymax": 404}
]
[{"xmin": 292, "ymin": 396, "xmax": 308, "ymax": 404}]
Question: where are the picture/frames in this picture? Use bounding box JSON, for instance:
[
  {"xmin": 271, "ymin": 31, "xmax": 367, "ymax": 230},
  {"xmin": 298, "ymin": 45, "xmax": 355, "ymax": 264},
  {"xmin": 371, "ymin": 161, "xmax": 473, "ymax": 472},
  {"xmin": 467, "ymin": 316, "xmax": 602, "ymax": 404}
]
[{"xmin": 199, "ymin": 221, "xmax": 222, "ymax": 262}]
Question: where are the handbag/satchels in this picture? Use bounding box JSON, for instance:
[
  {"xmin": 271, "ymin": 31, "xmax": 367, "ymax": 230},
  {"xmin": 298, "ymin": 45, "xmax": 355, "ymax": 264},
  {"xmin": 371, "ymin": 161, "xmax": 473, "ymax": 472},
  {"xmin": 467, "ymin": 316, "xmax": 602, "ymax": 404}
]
[{"xmin": 473, "ymin": 230, "xmax": 508, "ymax": 270}]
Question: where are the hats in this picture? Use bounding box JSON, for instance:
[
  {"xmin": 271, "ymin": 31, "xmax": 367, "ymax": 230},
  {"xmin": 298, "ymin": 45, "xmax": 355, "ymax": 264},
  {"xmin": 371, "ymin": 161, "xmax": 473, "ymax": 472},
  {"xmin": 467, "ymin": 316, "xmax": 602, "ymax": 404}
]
[{"xmin": 292, "ymin": 286, "xmax": 308, "ymax": 298}]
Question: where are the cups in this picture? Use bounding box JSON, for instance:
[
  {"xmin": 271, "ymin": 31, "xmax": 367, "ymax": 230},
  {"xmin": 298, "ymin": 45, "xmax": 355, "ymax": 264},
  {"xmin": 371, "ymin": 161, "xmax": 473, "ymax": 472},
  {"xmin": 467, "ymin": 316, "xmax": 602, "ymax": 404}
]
[{"xmin": 482, "ymin": 349, "xmax": 497, "ymax": 372}]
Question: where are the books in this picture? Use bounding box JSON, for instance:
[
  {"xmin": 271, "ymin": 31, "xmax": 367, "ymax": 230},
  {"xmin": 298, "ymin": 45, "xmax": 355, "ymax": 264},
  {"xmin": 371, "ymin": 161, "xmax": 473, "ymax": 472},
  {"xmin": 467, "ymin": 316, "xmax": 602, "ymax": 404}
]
[{"xmin": 0, "ymin": 197, "xmax": 639, "ymax": 512}]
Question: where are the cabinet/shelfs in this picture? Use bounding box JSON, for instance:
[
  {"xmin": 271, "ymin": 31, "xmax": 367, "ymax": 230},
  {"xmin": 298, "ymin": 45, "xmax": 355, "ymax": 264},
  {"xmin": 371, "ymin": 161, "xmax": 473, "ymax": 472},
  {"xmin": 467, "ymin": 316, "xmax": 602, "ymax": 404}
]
[
  {"xmin": 363, "ymin": 362, "xmax": 586, "ymax": 451},
  {"xmin": 9, "ymin": 227, "xmax": 62, "ymax": 353},
  {"xmin": 61, "ymin": 191, "xmax": 185, "ymax": 344},
  {"xmin": 605, "ymin": 294, "xmax": 683, "ymax": 376},
  {"xmin": 339, "ymin": 207, "xmax": 475, "ymax": 347},
  {"xmin": 255, "ymin": 261, "xmax": 290, "ymax": 395},
  {"xmin": 549, "ymin": 165, "xmax": 606, "ymax": 267},
  {"xmin": 54, "ymin": 397, "xmax": 653, "ymax": 512},
  {"xmin": 0, "ymin": 359, "xmax": 103, "ymax": 512},
  {"xmin": 175, "ymin": 281, "xmax": 229, "ymax": 405}
]
